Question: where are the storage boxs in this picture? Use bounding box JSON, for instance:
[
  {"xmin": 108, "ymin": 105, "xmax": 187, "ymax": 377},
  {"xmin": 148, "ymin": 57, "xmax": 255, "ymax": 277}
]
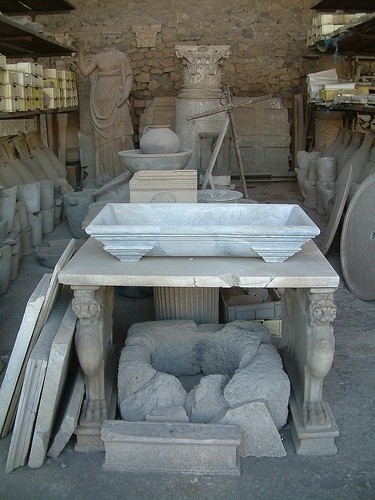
[{"xmin": 219, "ymin": 287, "xmax": 283, "ymax": 336}]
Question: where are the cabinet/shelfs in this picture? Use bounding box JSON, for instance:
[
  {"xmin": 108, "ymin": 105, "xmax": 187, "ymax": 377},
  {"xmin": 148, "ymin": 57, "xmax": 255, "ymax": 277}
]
[
  {"xmin": 306, "ymin": 0, "xmax": 375, "ymax": 180},
  {"xmin": 1, "ymin": 0, "xmax": 81, "ymax": 180}
]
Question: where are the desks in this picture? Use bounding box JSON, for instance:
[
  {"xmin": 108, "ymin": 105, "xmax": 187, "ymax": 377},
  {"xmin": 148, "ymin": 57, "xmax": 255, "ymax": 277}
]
[{"xmin": 57, "ymin": 236, "xmax": 340, "ymax": 457}]
